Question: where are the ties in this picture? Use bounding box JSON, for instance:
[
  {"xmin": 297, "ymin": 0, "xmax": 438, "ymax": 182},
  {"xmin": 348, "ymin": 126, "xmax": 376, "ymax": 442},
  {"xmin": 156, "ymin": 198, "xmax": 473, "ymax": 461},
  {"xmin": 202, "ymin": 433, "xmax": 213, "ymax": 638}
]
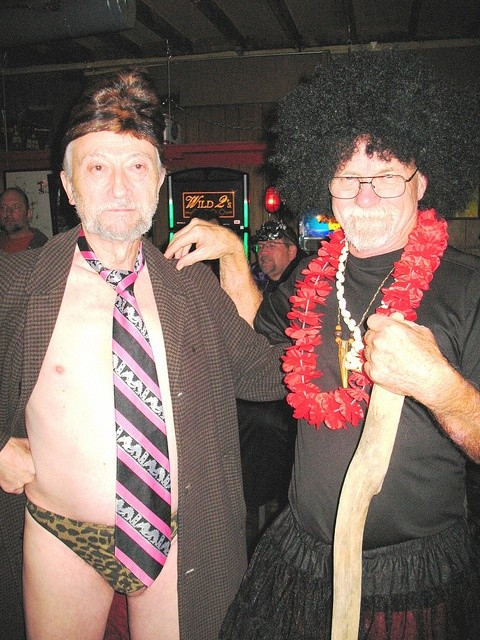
[{"xmin": 77, "ymin": 222, "xmax": 173, "ymax": 589}]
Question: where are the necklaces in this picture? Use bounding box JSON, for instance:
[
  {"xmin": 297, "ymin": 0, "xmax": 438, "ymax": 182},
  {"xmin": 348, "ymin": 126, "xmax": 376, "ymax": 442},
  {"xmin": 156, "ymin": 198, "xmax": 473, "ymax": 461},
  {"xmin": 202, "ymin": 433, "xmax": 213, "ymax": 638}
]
[
  {"xmin": 334, "ymin": 255, "xmax": 396, "ymax": 389},
  {"xmin": 335, "ymin": 242, "xmax": 398, "ymax": 405}
]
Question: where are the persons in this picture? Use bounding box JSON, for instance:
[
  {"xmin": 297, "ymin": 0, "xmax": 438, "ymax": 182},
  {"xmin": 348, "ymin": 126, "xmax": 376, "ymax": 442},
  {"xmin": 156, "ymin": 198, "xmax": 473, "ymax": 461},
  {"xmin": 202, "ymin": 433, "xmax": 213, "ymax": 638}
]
[
  {"xmin": 1, "ymin": 71, "xmax": 292, "ymax": 640},
  {"xmin": 165, "ymin": 50, "xmax": 480, "ymax": 640},
  {"xmin": 249, "ymin": 218, "xmax": 307, "ymax": 298},
  {"xmin": 0, "ymin": 186, "xmax": 49, "ymax": 252}
]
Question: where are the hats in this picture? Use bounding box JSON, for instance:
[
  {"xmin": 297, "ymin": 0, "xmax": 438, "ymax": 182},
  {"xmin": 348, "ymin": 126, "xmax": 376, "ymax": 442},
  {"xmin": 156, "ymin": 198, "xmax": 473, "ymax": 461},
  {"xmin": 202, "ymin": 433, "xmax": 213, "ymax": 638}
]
[{"xmin": 250, "ymin": 219, "xmax": 300, "ymax": 247}]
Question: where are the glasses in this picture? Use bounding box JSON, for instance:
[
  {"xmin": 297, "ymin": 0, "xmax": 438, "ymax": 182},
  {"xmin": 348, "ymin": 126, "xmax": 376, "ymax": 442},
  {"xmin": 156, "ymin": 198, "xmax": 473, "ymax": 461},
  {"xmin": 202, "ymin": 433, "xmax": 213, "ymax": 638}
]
[
  {"xmin": 327, "ymin": 163, "xmax": 419, "ymax": 200},
  {"xmin": 258, "ymin": 241, "xmax": 293, "ymax": 251}
]
